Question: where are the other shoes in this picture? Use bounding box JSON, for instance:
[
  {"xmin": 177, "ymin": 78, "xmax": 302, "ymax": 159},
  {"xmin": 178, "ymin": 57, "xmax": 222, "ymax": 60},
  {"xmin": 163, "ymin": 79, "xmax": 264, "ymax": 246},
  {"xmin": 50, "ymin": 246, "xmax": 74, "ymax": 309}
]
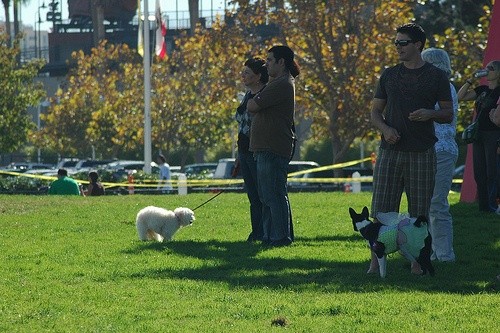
[{"xmin": 269, "ymin": 237, "xmax": 292, "ymax": 246}]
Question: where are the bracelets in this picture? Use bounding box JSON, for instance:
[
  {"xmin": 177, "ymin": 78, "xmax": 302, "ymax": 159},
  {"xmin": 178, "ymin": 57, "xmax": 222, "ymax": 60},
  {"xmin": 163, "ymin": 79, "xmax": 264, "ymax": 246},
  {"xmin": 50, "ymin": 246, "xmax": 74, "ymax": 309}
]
[{"xmin": 466, "ymin": 81, "xmax": 473, "ymax": 86}]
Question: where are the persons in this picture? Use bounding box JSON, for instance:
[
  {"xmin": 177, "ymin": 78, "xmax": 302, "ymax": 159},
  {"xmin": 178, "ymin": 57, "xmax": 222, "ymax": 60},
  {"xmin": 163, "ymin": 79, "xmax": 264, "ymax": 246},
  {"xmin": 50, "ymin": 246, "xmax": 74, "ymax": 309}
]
[
  {"xmin": 47, "ymin": 169, "xmax": 81, "ymax": 196},
  {"xmin": 81, "ymin": 172, "xmax": 105, "ymax": 196},
  {"xmin": 365, "ymin": 25, "xmax": 455, "ymax": 276},
  {"xmin": 235, "ymin": 58, "xmax": 295, "ymax": 242},
  {"xmin": 247, "ymin": 46, "xmax": 300, "ymax": 248},
  {"xmin": 157, "ymin": 156, "xmax": 173, "ymax": 194},
  {"xmin": 457, "ymin": 60, "xmax": 500, "ymax": 215},
  {"xmin": 421, "ymin": 48, "xmax": 457, "ymax": 264}
]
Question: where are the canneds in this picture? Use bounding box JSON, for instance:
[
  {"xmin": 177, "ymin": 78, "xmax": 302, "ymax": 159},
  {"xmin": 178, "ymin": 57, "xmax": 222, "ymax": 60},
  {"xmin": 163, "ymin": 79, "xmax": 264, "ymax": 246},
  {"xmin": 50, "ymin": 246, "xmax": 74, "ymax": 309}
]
[{"xmin": 474, "ymin": 70, "xmax": 488, "ymax": 77}]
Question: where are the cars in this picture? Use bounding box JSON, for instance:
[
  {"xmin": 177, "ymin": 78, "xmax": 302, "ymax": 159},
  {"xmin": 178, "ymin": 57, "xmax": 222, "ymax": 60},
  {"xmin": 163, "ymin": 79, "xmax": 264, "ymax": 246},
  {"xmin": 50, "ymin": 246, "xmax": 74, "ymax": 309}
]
[{"xmin": 8, "ymin": 158, "xmax": 319, "ymax": 183}]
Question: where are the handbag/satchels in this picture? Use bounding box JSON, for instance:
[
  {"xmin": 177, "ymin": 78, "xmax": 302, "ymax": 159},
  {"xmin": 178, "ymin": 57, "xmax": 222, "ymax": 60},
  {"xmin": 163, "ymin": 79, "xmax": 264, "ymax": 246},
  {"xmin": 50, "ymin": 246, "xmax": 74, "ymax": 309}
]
[{"xmin": 462, "ymin": 122, "xmax": 480, "ymax": 144}]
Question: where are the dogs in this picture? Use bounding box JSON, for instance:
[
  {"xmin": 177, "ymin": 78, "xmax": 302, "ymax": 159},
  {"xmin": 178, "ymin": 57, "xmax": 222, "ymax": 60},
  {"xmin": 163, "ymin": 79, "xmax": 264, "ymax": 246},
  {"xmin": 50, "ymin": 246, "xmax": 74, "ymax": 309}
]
[
  {"xmin": 136, "ymin": 206, "xmax": 196, "ymax": 242},
  {"xmin": 349, "ymin": 206, "xmax": 436, "ymax": 278}
]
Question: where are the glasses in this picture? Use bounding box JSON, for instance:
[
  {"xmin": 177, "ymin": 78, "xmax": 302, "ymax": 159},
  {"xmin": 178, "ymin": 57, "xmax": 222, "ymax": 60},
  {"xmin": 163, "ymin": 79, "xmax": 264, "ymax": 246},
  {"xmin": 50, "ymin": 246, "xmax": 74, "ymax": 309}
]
[
  {"xmin": 395, "ymin": 40, "xmax": 417, "ymax": 46},
  {"xmin": 487, "ymin": 65, "xmax": 497, "ymax": 71}
]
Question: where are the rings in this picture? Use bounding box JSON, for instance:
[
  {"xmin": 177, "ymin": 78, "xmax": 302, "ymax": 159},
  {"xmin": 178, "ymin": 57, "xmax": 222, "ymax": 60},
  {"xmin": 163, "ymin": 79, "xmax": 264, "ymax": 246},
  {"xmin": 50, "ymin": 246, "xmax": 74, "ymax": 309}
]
[{"xmin": 419, "ymin": 117, "xmax": 422, "ymax": 119}]
